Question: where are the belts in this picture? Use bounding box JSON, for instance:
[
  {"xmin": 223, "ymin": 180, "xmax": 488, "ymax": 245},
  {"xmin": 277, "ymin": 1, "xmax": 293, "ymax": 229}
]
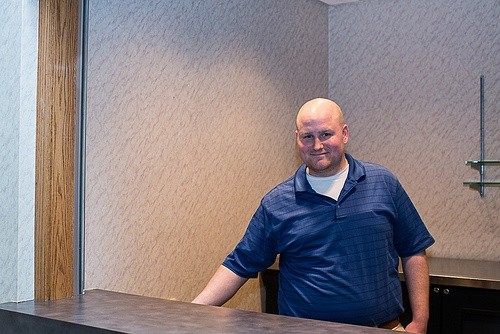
[{"xmin": 375, "ymin": 317, "xmax": 400, "ymax": 329}]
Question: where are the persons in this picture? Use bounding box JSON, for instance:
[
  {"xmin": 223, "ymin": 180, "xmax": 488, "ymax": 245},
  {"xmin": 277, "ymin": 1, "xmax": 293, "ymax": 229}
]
[{"xmin": 192, "ymin": 97, "xmax": 436, "ymax": 334}]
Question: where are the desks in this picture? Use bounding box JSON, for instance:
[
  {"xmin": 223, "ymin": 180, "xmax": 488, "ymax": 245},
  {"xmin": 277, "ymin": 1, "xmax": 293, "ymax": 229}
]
[
  {"xmin": 258, "ymin": 256, "xmax": 500, "ymax": 314},
  {"xmin": 0, "ymin": 289, "xmax": 418, "ymax": 334}
]
